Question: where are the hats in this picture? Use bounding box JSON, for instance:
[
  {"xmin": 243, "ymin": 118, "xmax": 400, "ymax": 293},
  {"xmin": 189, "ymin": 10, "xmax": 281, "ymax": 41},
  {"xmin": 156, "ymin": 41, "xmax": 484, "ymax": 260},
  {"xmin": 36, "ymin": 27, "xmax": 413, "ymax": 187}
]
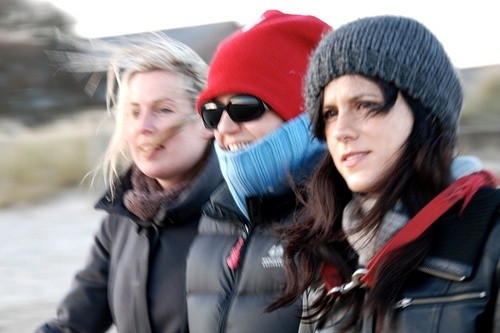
[
  {"xmin": 303, "ymin": 14, "xmax": 462, "ymax": 139},
  {"xmin": 195, "ymin": 10, "xmax": 334, "ymax": 122}
]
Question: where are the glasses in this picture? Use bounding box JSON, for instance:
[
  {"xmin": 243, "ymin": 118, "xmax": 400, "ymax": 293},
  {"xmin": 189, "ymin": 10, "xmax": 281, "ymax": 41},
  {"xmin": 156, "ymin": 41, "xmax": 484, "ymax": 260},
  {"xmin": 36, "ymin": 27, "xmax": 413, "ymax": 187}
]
[{"xmin": 200, "ymin": 94, "xmax": 270, "ymax": 128}]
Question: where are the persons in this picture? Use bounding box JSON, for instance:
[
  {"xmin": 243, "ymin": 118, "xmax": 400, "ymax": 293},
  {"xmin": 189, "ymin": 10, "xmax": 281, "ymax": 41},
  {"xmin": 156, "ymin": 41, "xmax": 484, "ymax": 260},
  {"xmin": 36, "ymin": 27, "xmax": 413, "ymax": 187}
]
[
  {"xmin": 186, "ymin": 10, "xmax": 333, "ymax": 333},
  {"xmin": 35, "ymin": 37, "xmax": 224, "ymax": 333},
  {"xmin": 266, "ymin": 15, "xmax": 500, "ymax": 333}
]
